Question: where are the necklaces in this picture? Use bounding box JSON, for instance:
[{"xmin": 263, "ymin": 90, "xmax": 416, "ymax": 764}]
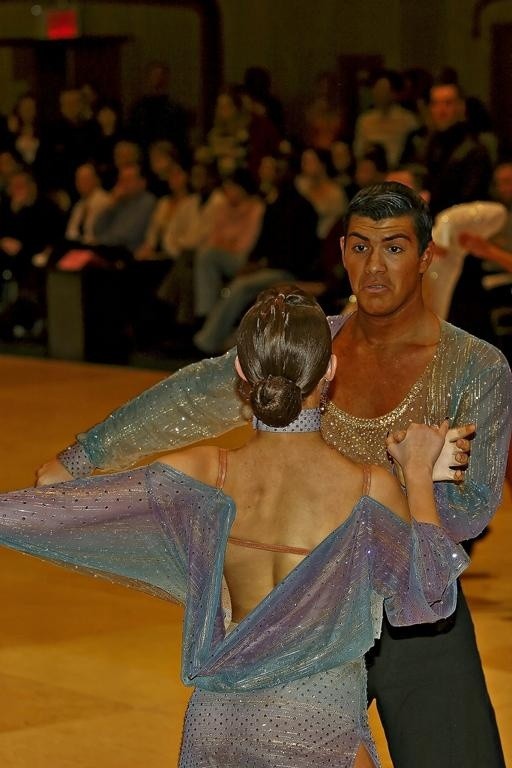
[{"xmin": 252, "ymin": 405, "xmax": 322, "ymax": 433}]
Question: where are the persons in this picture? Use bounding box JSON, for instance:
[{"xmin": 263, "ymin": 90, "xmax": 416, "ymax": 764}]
[
  {"xmin": 2, "ymin": 284, "xmax": 471, "ymax": 768},
  {"xmin": 29, "ymin": 180, "xmax": 511, "ymax": 768},
  {"xmin": 2, "ymin": 56, "xmax": 512, "ymax": 371}
]
